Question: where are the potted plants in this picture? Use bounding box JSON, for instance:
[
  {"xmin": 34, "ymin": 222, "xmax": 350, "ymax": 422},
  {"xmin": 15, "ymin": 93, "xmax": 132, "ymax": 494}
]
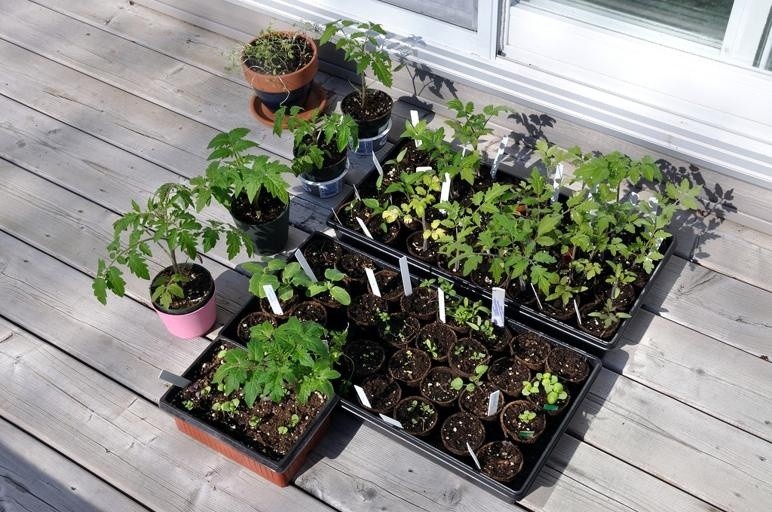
[
  {"xmin": 330, "ymin": 137, "xmax": 673, "ymax": 339},
  {"xmin": 268, "ymin": 97, "xmax": 361, "ymax": 200},
  {"xmin": 224, "ymin": 32, "xmax": 329, "ymax": 119},
  {"xmin": 319, "ymin": 23, "xmax": 405, "ymax": 152},
  {"xmin": 187, "ymin": 129, "xmax": 291, "ymax": 251},
  {"xmin": 93, "ymin": 183, "xmax": 226, "ymax": 340},
  {"xmin": 220, "ymin": 231, "xmax": 591, "ymax": 482},
  {"xmin": 152, "ymin": 336, "xmax": 340, "ymax": 486}
]
